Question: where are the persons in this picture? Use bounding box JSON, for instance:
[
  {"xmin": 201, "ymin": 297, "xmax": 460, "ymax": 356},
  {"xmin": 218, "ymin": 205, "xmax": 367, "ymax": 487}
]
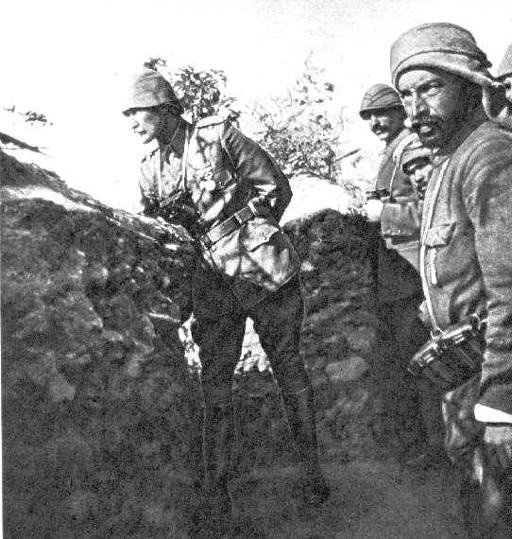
[{"xmin": 121, "ymin": 71, "xmax": 331, "ymax": 529}]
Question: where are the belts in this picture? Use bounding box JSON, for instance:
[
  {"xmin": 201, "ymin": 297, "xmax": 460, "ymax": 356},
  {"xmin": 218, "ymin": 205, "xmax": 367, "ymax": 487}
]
[{"xmin": 200, "ymin": 207, "xmax": 255, "ymax": 251}]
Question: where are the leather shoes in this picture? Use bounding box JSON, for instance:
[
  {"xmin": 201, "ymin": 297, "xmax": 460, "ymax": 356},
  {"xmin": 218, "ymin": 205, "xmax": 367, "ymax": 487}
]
[
  {"xmin": 299, "ymin": 476, "xmax": 330, "ymax": 508},
  {"xmin": 407, "ymin": 448, "xmax": 451, "ymax": 468}
]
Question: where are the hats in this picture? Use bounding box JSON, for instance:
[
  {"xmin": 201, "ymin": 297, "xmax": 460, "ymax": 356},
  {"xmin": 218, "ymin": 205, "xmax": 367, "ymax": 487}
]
[{"xmin": 388, "ymin": 22, "xmax": 493, "ymax": 83}]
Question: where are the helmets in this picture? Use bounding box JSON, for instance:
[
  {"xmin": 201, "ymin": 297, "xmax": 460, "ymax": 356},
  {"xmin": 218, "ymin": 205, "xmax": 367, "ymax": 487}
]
[
  {"xmin": 359, "ymin": 83, "xmax": 406, "ymax": 119},
  {"xmin": 495, "ymin": 43, "xmax": 512, "ymax": 78},
  {"xmin": 120, "ymin": 69, "xmax": 185, "ymax": 116}
]
